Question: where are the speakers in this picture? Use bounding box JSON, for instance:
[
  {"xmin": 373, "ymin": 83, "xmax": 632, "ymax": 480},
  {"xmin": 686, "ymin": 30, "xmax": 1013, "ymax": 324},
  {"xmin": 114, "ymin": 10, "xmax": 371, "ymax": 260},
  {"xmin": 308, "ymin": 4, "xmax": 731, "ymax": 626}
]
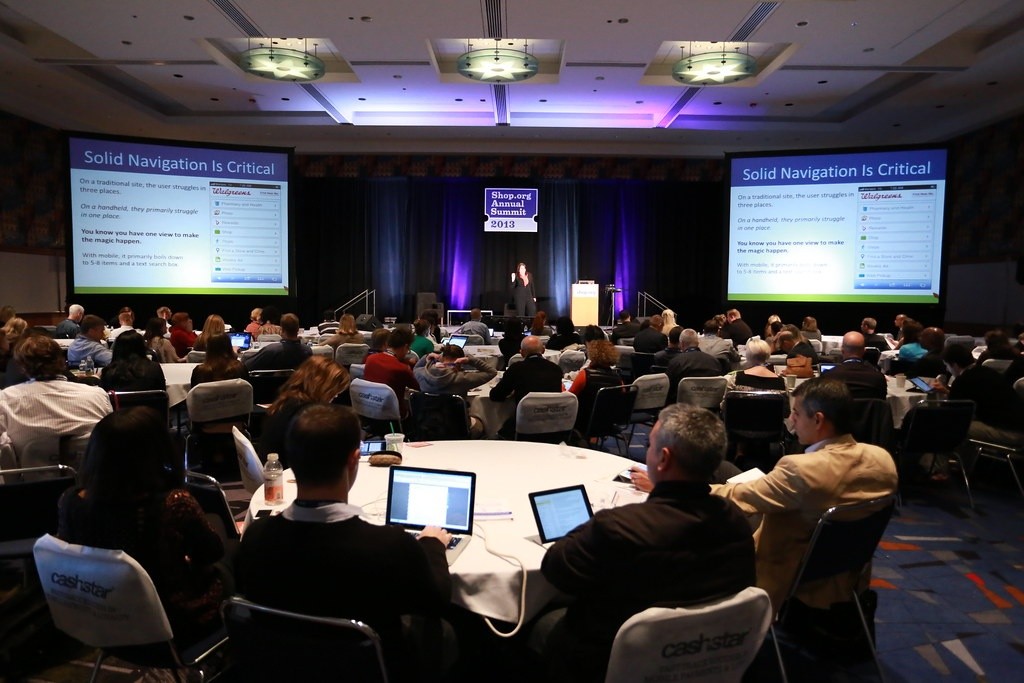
[
  {"xmin": 481, "ymin": 315, "xmax": 535, "ymax": 331},
  {"xmin": 356, "ymin": 314, "xmax": 384, "ymax": 332}
]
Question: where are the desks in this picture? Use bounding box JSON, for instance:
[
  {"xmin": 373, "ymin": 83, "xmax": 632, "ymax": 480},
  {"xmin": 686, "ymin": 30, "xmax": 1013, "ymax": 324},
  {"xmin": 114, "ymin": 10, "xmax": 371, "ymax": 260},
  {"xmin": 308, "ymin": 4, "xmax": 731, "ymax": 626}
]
[
  {"xmin": 249, "ymin": 440, "xmax": 682, "ymax": 625},
  {"xmin": 455, "ymin": 371, "xmax": 573, "ymax": 439},
  {"xmin": 463, "ymin": 344, "xmax": 561, "ymax": 372},
  {"xmin": 237, "ymin": 341, "xmax": 334, "ymax": 361},
  {"xmin": 50, "ymin": 339, "xmax": 107, "ymax": 351},
  {"xmin": 779, "ymin": 374, "xmax": 941, "ymax": 429},
  {"xmin": 259, "ymin": 331, "xmax": 320, "ymax": 344},
  {"xmin": 881, "ymin": 350, "xmax": 900, "ymax": 374},
  {"xmin": 93, "ymin": 363, "xmax": 205, "ymax": 432}
]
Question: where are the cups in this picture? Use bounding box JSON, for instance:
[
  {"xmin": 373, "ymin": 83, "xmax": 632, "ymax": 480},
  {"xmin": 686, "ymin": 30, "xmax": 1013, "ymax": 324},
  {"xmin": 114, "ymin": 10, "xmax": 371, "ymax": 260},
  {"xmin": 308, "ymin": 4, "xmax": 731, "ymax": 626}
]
[
  {"xmin": 786, "ymin": 375, "xmax": 797, "ymax": 388},
  {"xmin": 896, "ymin": 376, "xmax": 906, "ymax": 388},
  {"xmin": 384, "ymin": 433, "xmax": 405, "ymax": 452}
]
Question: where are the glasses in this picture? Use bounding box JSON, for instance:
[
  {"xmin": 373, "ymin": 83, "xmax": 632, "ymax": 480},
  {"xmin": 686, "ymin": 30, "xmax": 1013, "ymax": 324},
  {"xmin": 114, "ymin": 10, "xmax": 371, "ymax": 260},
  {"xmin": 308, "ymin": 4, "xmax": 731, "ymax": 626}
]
[{"xmin": 406, "ymin": 347, "xmax": 411, "ymax": 355}]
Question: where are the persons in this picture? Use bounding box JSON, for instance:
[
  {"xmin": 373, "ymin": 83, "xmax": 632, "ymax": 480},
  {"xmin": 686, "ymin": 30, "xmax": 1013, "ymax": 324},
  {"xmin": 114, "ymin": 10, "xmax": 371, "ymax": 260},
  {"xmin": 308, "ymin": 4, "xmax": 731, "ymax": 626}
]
[{"xmin": 0, "ymin": 262, "xmax": 1024, "ymax": 683}]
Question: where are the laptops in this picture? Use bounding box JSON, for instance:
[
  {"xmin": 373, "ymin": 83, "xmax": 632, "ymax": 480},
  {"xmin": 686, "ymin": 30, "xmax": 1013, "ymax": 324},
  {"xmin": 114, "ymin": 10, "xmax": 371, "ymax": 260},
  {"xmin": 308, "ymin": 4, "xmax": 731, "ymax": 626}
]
[
  {"xmin": 447, "ymin": 334, "xmax": 470, "ymax": 349},
  {"xmin": 227, "ymin": 332, "xmax": 252, "ymax": 351},
  {"xmin": 385, "ymin": 465, "xmax": 476, "ymax": 565}
]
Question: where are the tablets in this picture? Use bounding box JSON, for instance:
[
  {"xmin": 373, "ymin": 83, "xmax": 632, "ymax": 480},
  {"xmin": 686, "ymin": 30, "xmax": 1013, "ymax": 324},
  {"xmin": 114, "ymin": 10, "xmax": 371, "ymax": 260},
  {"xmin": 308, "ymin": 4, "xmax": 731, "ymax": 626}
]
[
  {"xmin": 819, "ymin": 363, "xmax": 836, "ymax": 374},
  {"xmin": 359, "ymin": 441, "xmax": 386, "ymax": 455},
  {"xmin": 908, "ymin": 377, "xmax": 932, "ymax": 392},
  {"xmin": 528, "ymin": 484, "xmax": 594, "ymax": 544}
]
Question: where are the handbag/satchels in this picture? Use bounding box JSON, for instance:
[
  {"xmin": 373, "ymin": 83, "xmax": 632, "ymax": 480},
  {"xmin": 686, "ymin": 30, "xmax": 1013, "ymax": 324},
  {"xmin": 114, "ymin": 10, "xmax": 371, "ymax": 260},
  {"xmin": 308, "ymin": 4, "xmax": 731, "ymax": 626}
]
[{"xmin": 783, "ymin": 354, "xmax": 814, "ymax": 378}]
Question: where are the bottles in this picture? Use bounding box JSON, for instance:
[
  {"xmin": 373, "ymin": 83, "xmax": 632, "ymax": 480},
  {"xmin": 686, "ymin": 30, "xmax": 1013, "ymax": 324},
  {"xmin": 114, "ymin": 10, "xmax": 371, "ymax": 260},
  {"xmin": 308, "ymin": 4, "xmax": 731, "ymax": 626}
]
[
  {"xmin": 524, "ymin": 325, "xmax": 528, "ymax": 332},
  {"xmin": 262, "ymin": 453, "xmax": 284, "ymax": 506},
  {"xmin": 306, "ymin": 339, "xmax": 314, "ymax": 348},
  {"xmin": 79, "ymin": 356, "xmax": 95, "ymax": 379},
  {"xmin": 254, "ymin": 343, "xmax": 259, "ymax": 349},
  {"xmin": 110, "ymin": 326, "xmax": 114, "ymax": 331}
]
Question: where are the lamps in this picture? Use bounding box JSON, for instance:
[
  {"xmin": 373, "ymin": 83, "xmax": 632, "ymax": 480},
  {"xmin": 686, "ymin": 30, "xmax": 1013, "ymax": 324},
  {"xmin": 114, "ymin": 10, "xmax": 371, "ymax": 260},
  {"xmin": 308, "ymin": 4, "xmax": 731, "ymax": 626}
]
[
  {"xmin": 240, "ymin": 38, "xmax": 326, "ymax": 82},
  {"xmin": 456, "ymin": 38, "xmax": 539, "ymax": 83},
  {"xmin": 672, "ymin": 41, "xmax": 758, "ymax": 85},
  {"xmin": 356, "ymin": 314, "xmax": 384, "ymax": 331}
]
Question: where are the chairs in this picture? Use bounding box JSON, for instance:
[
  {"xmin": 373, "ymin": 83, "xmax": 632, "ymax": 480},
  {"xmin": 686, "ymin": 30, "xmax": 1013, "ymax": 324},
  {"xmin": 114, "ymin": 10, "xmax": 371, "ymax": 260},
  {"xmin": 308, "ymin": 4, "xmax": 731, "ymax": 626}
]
[
  {"xmin": 417, "ymin": 293, "xmax": 443, "ymax": 326},
  {"xmin": 0, "ymin": 334, "xmax": 1024, "ymax": 683},
  {"xmin": 504, "ymin": 297, "xmax": 518, "ymax": 316}
]
